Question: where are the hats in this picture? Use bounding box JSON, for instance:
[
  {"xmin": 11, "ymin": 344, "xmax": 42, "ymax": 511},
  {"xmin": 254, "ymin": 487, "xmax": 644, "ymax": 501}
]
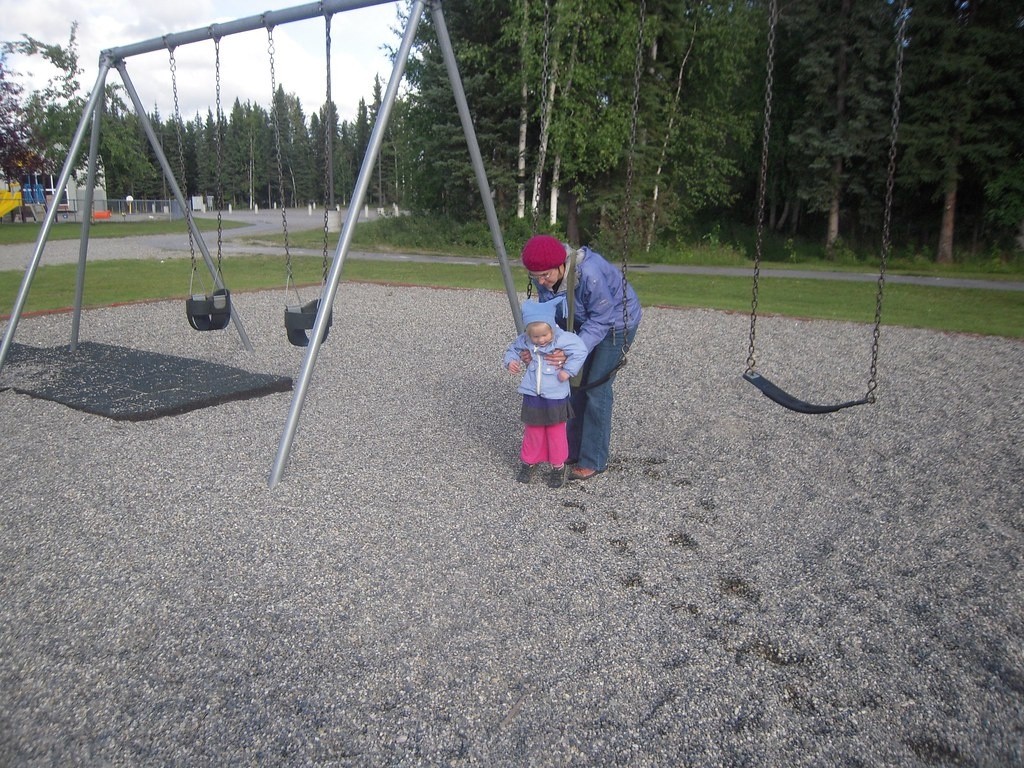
[
  {"xmin": 521, "ymin": 296, "xmax": 564, "ymax": 330},
  {"xmin": 521, "ymin": 235, "xmax": 567, "ymax": 271}
]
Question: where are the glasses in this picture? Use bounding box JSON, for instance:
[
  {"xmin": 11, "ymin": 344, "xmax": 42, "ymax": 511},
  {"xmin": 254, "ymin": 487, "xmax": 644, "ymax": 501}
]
[{"xmin": 528, "ymin": 270, "xmax": 552, "ymax": 279}]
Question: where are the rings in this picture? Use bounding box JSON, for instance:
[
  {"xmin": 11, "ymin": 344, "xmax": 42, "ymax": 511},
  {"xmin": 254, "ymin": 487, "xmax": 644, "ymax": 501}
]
[
  {"xmin": 558, "ymin": 364, "xmax": 562, "ymax": 368},
  {"xmin": 558, "ymin": 361, "xmax": 561, "ymax": 365}
]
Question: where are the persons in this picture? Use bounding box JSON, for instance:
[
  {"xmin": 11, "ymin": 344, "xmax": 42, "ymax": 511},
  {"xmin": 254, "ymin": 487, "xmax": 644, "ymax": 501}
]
[
  {"xmin": 517, "ymin": 236, "xmax": 643, "ymax": 481},
  {"xmin": 504, "ymin": 295, "xmax": 589, "ymax": 490}
]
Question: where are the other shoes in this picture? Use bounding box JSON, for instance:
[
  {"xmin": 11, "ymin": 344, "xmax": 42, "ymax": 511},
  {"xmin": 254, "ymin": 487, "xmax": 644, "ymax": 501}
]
[{"xmin": 569, "ymin": 465, "xmax": 597, "ymax": 479}]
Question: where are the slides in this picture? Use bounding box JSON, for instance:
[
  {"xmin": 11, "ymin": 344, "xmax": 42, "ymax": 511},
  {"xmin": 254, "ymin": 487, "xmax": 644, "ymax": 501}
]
[{"xmin": 0, "ymin": 192, "xmax": 22, "ymax": 217}]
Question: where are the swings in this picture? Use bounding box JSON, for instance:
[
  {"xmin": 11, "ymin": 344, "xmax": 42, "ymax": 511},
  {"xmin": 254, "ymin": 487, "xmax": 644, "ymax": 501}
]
[
  {"xmin": 739, "ymin": 1, "xmax": 908, "ymax": 416},
  {"xmin": 169, "ymin": 41, "xmax": 231, "ymax": 331},
  {"xmin": 269, "ymin": 16, "xmax": 333, "ymax": 345},
  {"xmin": 527, "ymin": 0, "xmax": 645, "ymax": 391}
]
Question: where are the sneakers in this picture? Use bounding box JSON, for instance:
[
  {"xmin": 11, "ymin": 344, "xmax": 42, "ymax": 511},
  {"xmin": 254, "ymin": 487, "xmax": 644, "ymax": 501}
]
[
  {"xmin": 516, "ymin": 463, "xmax": 535, "ymax": 483},
  {"xmin": 548, "ymin": 465, "xmax": 566, "ymax": 489}
]
